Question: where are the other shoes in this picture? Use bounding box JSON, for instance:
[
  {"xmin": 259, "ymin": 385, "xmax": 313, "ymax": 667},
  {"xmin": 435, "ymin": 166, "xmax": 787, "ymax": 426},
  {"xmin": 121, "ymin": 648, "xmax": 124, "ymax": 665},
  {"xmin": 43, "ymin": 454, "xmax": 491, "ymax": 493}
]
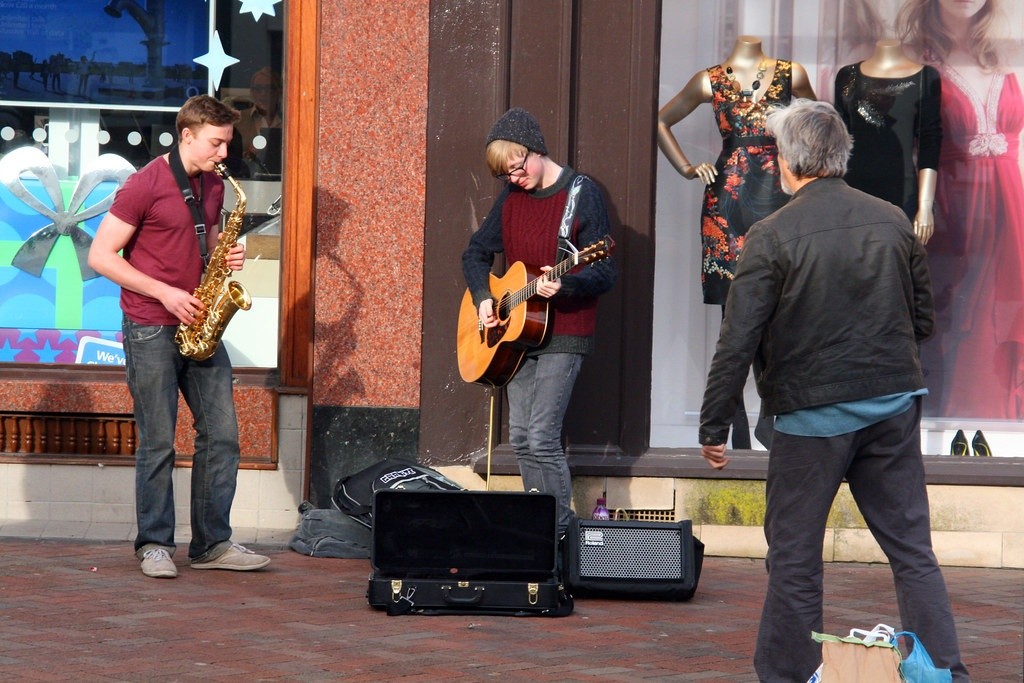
[
  {"xmin": 950, "ymin": 429, "xmax": 968, "ymax": 456},
  {"xmin": 971, "ymin": 429, "xmax": 991, "ymax": 457}
]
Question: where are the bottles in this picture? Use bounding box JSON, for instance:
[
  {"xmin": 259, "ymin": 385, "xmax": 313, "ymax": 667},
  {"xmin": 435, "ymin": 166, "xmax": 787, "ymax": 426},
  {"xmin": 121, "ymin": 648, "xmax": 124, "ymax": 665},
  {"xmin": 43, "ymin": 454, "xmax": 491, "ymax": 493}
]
[{"xmin": 592, "ymin": 497, "xmax": 610, "ymax": 520}]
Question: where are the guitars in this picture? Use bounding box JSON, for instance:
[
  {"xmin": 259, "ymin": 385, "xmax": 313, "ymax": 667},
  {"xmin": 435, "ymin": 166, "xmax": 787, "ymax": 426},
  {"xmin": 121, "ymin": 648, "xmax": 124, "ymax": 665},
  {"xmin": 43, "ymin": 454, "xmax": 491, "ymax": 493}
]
[{"xmin": 457, "ymin": 234, "xmax": 617, "ymax": 390}]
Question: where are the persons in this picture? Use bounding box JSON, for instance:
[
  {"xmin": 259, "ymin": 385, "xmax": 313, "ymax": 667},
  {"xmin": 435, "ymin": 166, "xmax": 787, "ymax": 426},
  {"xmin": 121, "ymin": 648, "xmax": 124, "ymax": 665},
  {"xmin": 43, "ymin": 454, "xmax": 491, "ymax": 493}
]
[
  {"xmin": 0, "ymin": 54, "xmax": 181, "ymax": 99},
  {"xmin": 656, "ymin": 34, "xmax": 817, "ymax": 450},
  {"xmin": 87, "ymin": 95, "xmax": 272, "ymax": 578},
  {"xmin": 698, "ymin": 98, "xmax": 975, "ymax": 683},
  {"xmin": 895, "ymin": 0, "xmax": 1024, "ymax": 418},
  {"xmin": 834, "ymin": 38, "xmax": 942, "ymax": 247},
  {"xmin": 460, "ymin": 109, "xmax": 622, "ymax": 575},
  {"xmin": 215, "ymin": 65, "xmax": 282, "ymax": 181}
]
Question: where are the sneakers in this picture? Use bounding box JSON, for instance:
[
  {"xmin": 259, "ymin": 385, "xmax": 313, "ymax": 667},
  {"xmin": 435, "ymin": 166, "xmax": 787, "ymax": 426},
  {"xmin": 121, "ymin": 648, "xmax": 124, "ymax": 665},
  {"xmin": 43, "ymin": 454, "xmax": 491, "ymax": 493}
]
[
  {"xmin": 191, "ymin": 542, "xmax": 270, "ymax": 571},
  {"xmin": 140, "ymin": 548, "xmax": 176, "ymax": 577}
]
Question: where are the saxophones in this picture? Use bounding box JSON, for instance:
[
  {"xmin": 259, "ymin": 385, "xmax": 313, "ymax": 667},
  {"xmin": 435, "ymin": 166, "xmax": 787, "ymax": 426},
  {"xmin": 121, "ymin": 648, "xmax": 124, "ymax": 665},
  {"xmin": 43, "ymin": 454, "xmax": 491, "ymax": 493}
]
[{"xmin": 173, "ymin": 162, "xmax": 253, "ymax": 362}]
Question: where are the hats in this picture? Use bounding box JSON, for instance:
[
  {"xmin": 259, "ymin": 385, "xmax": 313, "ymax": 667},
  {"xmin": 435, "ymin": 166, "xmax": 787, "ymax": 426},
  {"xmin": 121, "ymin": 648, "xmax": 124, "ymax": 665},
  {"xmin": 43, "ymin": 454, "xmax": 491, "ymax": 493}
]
[{"xmin": 486, "ymin": 108, "xmax": 548, "ymax": 154}]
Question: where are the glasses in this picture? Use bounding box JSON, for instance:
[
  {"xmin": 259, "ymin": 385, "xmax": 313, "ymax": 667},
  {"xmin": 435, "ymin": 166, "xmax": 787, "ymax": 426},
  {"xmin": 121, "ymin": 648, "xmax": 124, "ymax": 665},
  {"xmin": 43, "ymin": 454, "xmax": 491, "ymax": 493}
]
[{"xmin": 494, "ymin": 149, "xmax": 530, "ymax": 180}]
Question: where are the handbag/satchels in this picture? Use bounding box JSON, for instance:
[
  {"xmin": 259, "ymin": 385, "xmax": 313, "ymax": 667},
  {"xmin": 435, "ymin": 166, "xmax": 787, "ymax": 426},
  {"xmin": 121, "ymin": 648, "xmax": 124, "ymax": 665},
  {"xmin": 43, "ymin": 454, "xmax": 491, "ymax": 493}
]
[
  {"xmin": 332, "ymin": 456, "xmax": 469, "ymax": 528},
  {"xmin": 803, "ymin": 624, "xmax": 952, "ymax": 683}
]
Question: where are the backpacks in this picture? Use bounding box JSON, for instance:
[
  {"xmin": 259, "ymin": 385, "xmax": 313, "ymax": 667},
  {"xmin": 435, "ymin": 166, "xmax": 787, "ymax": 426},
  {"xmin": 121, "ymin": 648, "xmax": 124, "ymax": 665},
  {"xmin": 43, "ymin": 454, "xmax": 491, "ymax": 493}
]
[{"xmin": 290, "ymin": 507, "xmax": 374, "ymax": 559}]
